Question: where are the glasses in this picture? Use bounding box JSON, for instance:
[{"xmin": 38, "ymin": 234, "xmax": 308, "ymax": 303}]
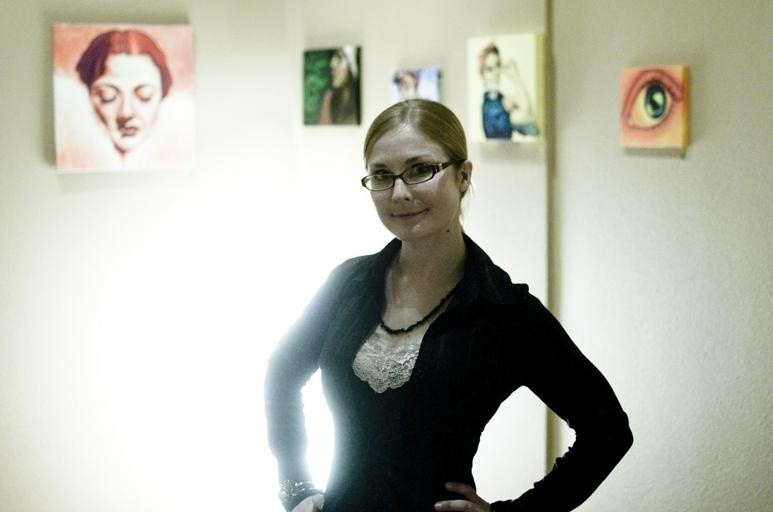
[{"xmin": 360, "ymin": 159, "xmax": 453, "ymax": 192}]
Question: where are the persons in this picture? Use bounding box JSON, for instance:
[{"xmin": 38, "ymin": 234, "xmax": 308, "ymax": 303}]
[
  {"xmin": 319, "ymin": 48, "xmax": 361, "ymax": 124},
  {"xmin": 475, "ymin": 41, "xmax": 533, "ymax": 138},
  {"xmin": 261, "ymin": 98, "xmax": 634, "ymax": 511},
  {"xmin": 391, "ymin": 67, "xmax": 418, "ymax": 100},
  {"xmin": 617, "ymin": 64, "xmax": 690, "ymax": 151},
  {"xmin": 74, "ymin": 28, "xmax": 172, "ymax": 154}
]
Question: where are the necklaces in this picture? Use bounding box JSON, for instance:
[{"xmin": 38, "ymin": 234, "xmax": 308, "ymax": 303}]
[{"xmin": 376, "ymin": 277, "xmax": 461, "ymax": 333}]
